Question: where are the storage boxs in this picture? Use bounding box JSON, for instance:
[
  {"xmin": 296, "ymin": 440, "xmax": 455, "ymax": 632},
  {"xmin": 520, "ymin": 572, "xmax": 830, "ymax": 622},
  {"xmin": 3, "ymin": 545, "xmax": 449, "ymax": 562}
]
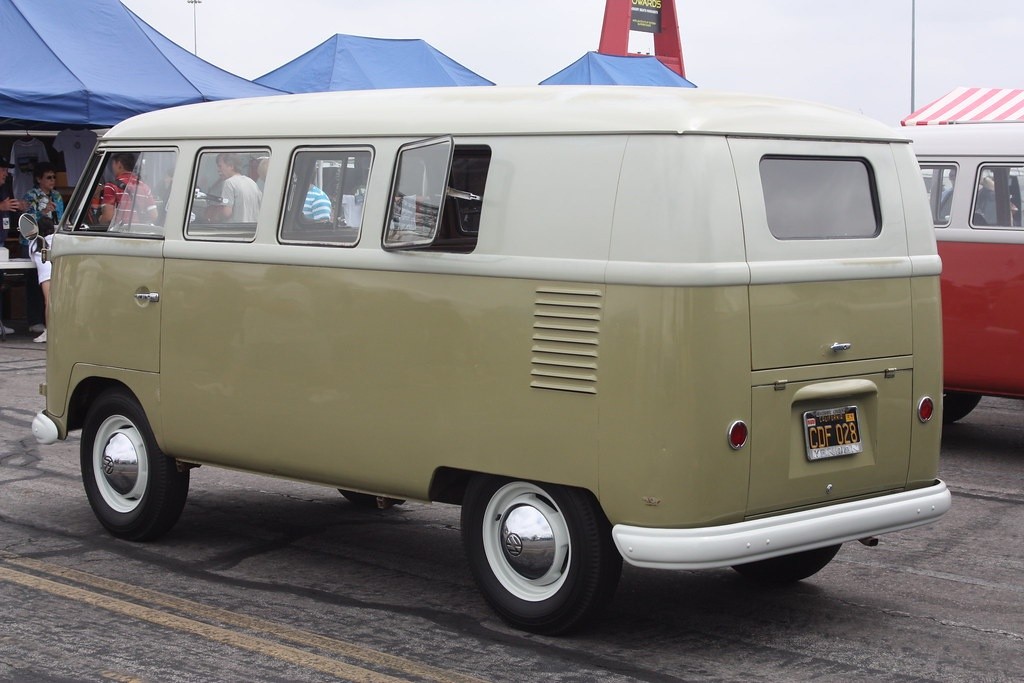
[{"xmin": 0, "ymin": 258, "xmax": 43, "ymax": 338}]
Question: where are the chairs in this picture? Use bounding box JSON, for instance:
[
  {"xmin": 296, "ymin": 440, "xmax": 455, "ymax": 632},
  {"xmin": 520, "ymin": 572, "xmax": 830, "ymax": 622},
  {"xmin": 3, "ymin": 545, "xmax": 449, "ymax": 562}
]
[
  {"xmin": 938, "ymin": 187, "xmax": 1013, "ymax": 226},
  {"xmin": 187, "ymin": 223, "xmax": 477, "ymax": 254}
]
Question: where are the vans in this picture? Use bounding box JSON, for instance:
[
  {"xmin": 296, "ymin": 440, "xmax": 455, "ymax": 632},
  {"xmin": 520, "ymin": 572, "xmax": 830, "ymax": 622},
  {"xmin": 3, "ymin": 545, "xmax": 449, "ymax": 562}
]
[
  {"xmin": 18, "ymin": 85, "xmax": 955, "ymax": 639},
  {"xmin": 898, "ymin": 123, "xmax": 1024, "ymax": 432}
]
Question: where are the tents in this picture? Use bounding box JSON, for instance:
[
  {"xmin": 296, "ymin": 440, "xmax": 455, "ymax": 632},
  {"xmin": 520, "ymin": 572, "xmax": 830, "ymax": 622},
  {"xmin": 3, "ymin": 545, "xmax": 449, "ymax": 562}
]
[
  {"xmin": 0, "ymin": 0, "xmax": 289, "ymax": 119},
  {"xmin": 898, "ymin": 86, "xmax": 1024, "ymax": 123},
  {"xmin": 538, "ymin": 52, "xmax": 697, "ymax": 89},
  {"xmin": 251, "ymin": 33, "xmax": 499, "ymax": 93}
]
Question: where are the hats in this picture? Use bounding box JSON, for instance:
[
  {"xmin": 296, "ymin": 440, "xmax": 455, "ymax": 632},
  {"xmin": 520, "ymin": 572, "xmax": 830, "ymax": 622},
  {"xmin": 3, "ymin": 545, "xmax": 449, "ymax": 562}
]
[{"xmin": 0, "ymin": 155, "xmax": 15, "ymax": 169}]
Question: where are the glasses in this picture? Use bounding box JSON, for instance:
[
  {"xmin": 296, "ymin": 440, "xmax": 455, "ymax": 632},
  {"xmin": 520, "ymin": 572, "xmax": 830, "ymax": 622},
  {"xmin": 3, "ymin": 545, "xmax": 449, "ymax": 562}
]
[{"xmin": 47, "ymin": 176, "xmax": 56, "ymax": 178}]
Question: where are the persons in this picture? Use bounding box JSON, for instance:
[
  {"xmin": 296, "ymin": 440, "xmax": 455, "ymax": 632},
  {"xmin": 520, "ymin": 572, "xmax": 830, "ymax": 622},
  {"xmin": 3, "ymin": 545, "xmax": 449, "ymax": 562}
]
[
  {"xmin": 96, "ymin": 151, "xmax": 159, "ymax": 229},
  {"xmin": 288, "ymin": 162, "xmax": 334, "ymax": 227},
  {"xmin": 0, "ymin": 156, "xmax": 74, "ymax": 344},
  {"xmin": 203, "ymin": 152, "xmax": 263, "ymax": 225},
  {"xmin": 931, "ymin": 163, "xmax": 1018, "ymax": 226}
]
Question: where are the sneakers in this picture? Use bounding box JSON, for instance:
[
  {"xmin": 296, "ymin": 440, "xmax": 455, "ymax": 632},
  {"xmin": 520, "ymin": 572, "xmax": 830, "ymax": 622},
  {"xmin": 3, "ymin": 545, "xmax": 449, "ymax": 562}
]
[{"xmin": 0, "ymin": 324, "xmax": 47, "ymax": 343}]
